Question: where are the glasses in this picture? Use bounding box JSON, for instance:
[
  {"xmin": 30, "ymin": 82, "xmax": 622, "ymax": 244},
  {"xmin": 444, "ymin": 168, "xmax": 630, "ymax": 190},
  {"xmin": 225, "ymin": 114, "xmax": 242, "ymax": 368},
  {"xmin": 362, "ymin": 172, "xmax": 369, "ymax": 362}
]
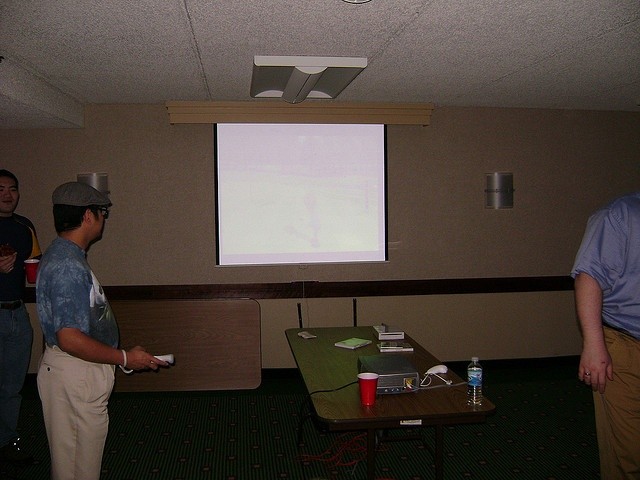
[{"xmin": 86, "ymin": 206, "xmax": 110, "ymax": 219}]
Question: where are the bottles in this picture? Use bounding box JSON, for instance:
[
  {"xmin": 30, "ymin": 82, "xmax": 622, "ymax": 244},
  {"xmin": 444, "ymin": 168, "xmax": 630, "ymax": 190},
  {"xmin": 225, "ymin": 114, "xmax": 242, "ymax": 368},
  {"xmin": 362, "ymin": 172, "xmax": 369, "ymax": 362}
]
[{"xmin": 468, "ymin": 357, "xmax": 484, "ymax": 406}]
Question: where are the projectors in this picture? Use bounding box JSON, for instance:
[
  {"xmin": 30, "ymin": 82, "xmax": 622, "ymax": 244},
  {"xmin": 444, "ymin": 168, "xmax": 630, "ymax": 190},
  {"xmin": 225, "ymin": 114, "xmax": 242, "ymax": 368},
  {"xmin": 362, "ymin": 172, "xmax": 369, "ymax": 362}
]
[{"xmin": 359, "ymin": 354, "xmax": 420, "ymax": 395}]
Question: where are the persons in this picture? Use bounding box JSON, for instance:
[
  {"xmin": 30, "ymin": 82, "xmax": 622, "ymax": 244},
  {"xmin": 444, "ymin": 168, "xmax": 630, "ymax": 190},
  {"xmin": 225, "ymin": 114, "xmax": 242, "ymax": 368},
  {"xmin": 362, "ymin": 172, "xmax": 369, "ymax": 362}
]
[
  {"xmin": 1, "ymin": 171, "xmax": 43, "ymax": 460},
  {"xmin": 35, "ymin": 181, "xmax": 169, "ymax": 480},
  {"xmin": 571, "ymin": 193, "xmax": 640, "ymax": 480}
]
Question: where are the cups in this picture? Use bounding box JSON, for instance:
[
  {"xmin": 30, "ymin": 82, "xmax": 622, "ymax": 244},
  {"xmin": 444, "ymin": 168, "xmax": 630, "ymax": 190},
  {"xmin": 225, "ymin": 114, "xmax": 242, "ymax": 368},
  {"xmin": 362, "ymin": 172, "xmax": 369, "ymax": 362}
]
[{"xmin": 357, "ymin": 373, "xmax": 379, "ymax": 405}]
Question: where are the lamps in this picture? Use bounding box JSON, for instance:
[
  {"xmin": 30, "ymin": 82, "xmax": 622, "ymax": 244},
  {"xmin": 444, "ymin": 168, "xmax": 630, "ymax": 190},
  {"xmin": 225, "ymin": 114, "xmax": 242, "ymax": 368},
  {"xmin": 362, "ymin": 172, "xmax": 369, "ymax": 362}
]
[
  {"xmin": 484, "ymin": 171, "xmax": 515, "ymax": 210},
  {"xmin": 250, "ymin": 55, "xmax": 368, "ymax": 103},
  {"xmin": 75, "ymin": 171, "xmax": 110, "ymax": 200}
]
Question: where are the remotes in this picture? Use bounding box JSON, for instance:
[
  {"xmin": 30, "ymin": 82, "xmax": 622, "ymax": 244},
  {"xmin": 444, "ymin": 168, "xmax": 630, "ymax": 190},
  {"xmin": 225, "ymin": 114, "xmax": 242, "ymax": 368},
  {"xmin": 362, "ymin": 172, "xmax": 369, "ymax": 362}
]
[{"xmin": 152, "ymin": 354, "xmax": 173, "ymax": 365}]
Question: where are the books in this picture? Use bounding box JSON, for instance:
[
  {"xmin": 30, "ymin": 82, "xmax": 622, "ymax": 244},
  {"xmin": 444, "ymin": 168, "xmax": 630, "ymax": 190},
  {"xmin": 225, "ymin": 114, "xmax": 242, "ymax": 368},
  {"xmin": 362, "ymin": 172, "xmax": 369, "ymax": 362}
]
[{"xmin": 334, "ymin": 338, "xmax": 373, "ymax": 349}]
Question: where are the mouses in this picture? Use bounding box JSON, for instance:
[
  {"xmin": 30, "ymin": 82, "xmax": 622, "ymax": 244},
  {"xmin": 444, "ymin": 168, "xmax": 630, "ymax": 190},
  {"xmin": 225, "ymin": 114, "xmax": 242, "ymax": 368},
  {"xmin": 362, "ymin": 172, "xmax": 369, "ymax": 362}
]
[{"xmin": 426, "ymin": 364, "xmax": 448, "ymax": 375}]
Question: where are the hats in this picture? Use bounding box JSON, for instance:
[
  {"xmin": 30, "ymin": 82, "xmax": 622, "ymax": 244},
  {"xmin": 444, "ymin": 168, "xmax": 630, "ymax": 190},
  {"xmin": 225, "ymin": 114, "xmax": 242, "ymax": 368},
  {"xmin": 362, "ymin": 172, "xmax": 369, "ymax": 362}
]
[{"xmin": 52, "ymin": 182, "xmax": 111, "ymax": 207}]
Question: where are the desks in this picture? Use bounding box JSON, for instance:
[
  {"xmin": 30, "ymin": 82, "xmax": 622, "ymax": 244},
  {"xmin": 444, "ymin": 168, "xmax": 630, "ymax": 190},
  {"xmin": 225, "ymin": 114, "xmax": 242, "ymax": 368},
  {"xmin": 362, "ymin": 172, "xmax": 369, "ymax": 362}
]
[
  {"xmin": 107, "ymin": 299, "xmax": 261, "ymax": 391},
  {"xmin": 284, "ymin": 325, "xmax": 496, "ymax": 478}
]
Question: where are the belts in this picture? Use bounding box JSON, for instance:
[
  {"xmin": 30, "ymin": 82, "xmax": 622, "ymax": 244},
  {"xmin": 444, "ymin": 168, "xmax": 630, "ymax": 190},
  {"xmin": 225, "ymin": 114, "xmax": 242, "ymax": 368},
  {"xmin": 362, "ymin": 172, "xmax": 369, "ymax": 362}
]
[{"xmin": 0, "ymin": 302, "xmax": 22, "ymax": 310}]
